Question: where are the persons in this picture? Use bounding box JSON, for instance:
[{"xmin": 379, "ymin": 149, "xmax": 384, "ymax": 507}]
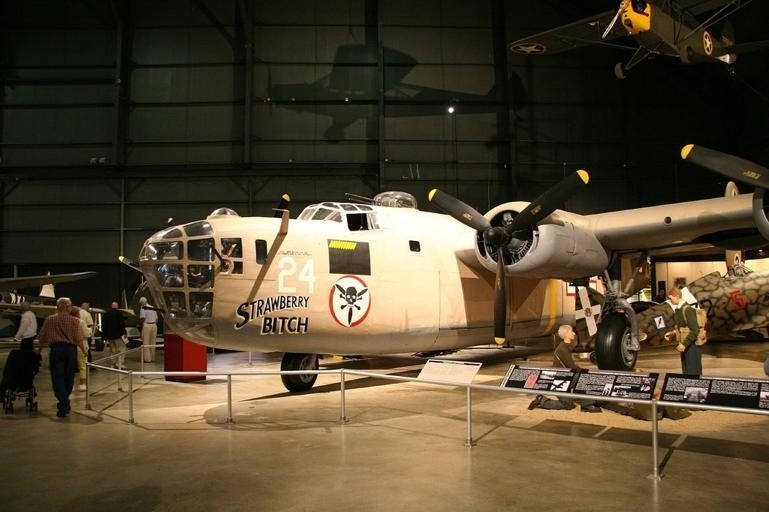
[
  {"xmin": 37, "ymin": 297, "xmax": 89, "ymax": 417},
  {"xmin": 137, "ymin": 296, "xmax": 158, "ymax": 362},
  {"xmin": 79, "ymin": 301, "xmax": 97, "ymax": 371},
  {"xmin": 71, "ymin": 306, "xmax": 93, "ymax": 392},
  {"xmin": 527, "ymin": 324, "xmax": 603, "ymax": 413},
  {"xmin": 664, "ymin": 285, "xmax": 705, "ymax": 381},
  {"xmin": 13, "ymin": 301, "xmax": 37, "ymax": 350},
  {"xmin": 102, "ymin": 302, "xmax": 128, "ymax": 369}
]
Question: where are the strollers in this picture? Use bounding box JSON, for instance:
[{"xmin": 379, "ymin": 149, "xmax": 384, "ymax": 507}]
[{"xmin": 1, "ymin": 348, "xmax": 41, "ymax": 414}]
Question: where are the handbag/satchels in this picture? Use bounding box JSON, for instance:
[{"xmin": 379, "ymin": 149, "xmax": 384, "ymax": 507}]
[{"xmin": 678, "ymin": 326, "xmax": 706, "ymax": 345}]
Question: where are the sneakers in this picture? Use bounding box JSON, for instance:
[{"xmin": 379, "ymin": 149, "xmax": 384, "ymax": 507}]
[
  {"xmin": 528, "ymin": 394, "xmax": 543, "ymax": 410},
  {"xmin": 581, "ymin": 404, "xmax": 601, "ymax": 413},
  {"xmin": 111, "ymin": 362, "xmax": 127, "ymax": 370},
  {"xmin": 78, "ymin": 384, "xmax": 86, "ymax": 392}
]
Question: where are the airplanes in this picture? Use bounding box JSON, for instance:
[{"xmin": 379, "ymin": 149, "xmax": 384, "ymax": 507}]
[
  {"xmin": 264, "ymin": 40, "xmax": 529, "ymax": 145},
  {"xmin": 118, "ymin": 141, "xmax": 769, "ymax": 396},
  {"xmin": 508, "ymin": 1, "xmax": 760, "ymax": 80},
  {"xmin": 565, "ymin": 262, "xmax": 769, "ymax": 366},
  {"xmin": 0, "ymin": 269, "xmax": 138, "ymax": 353}
]
